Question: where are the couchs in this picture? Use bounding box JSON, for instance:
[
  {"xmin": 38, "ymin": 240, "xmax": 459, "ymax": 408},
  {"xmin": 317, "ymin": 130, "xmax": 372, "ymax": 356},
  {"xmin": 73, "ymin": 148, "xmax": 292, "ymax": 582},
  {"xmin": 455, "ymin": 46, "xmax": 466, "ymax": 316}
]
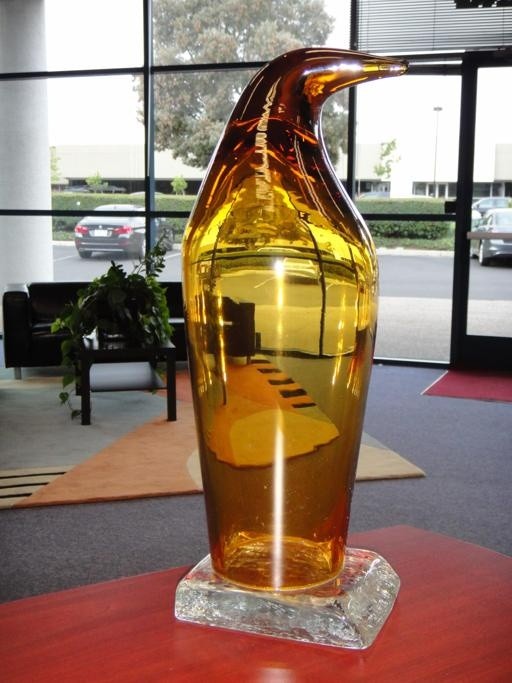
[{"xmin": 1, "ymin": 279, "xmax": 261, "ymax": 381}]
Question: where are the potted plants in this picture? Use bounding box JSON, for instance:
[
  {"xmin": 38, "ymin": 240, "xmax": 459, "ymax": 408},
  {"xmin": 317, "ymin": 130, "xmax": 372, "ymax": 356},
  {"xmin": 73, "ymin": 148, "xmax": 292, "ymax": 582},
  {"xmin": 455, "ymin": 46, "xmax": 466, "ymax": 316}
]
[{"xmin": 54, "ymin": 229, "xmax": 173, "ymax": 418}]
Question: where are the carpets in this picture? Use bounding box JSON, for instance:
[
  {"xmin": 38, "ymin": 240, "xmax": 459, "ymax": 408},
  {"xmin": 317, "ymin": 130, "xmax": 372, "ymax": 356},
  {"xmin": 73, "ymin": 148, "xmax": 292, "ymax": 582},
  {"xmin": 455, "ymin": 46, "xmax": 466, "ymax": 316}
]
[
  {"xmin": 0, "ymin": 374, "xmax": 428, "ymax": 509},
  {"xmin": 200, "ymin": 351, "xmax": 343, "ymax": 474},
  {"xmin": 418, "ymin": 368, "xmax": 511, "ymax": 402}
]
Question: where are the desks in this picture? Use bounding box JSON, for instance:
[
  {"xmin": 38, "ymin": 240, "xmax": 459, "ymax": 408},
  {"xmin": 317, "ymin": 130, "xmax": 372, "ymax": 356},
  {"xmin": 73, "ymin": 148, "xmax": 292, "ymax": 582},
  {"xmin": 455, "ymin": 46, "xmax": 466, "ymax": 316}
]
[{"xmin": 1, "ymin": 525, "xmax": 512, "ymax": 681}]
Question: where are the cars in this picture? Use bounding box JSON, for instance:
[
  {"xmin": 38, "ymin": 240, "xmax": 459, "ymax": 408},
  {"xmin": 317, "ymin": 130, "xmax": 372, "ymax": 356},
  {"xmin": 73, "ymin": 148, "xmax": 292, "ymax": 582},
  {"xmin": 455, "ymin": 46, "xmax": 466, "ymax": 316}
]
[
  {"xmin": 466, "ymin": 207, "xmax": 512, "ymax": 266},
  {"xmin": 472, "ymin": 194, "xmax": 512, "ymax": 225},
  {"xmin": 357, "ymin": 190, "xmax": 390, "ymax": 199},
  {"xmin": 73, "ymin": 201, "xmax": 178, "ymax": 262}
]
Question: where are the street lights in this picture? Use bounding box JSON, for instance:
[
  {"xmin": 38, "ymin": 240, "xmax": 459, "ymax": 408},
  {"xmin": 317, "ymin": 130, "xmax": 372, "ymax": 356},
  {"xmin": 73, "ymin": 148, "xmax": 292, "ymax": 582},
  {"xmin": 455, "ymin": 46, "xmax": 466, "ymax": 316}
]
[{"xmin": 431, "ymin": 104, "xmax": 447, "ymax": 198}]
